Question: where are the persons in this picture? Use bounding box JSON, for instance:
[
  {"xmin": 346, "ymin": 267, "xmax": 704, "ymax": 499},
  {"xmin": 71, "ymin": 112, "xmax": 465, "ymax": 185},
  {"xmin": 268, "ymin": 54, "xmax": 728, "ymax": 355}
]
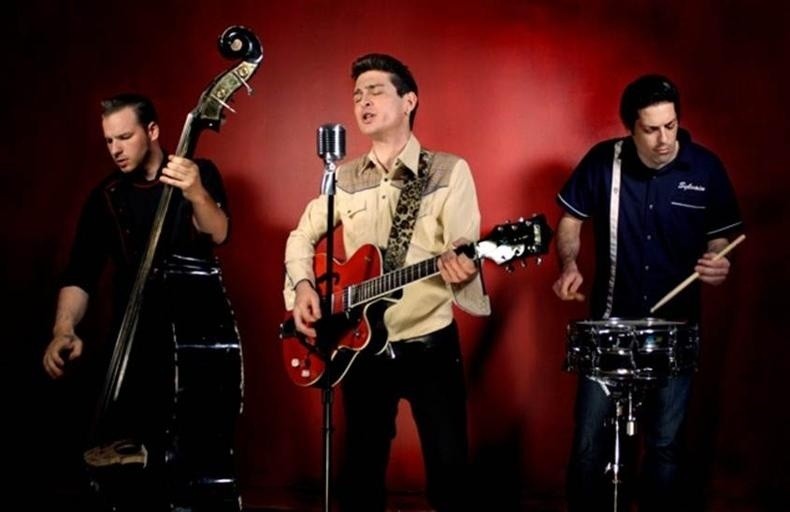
[
  {"xmin": 552, "ymin": 73, "xmax": 745, "ymax": 511},
  {"xmin": 282, "ymin": 52, "xmax": 492, "ymax": 512},
  {"xmin": 42, "ymin": 93, "xmax": 246, "ymax": 511}
]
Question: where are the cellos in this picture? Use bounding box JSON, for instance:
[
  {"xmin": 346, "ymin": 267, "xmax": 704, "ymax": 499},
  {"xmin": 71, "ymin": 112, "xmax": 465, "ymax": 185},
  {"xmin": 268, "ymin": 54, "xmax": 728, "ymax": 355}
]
[{"xmin": 47, "ymin": 24, "xmax": 264, "ymax": 512}]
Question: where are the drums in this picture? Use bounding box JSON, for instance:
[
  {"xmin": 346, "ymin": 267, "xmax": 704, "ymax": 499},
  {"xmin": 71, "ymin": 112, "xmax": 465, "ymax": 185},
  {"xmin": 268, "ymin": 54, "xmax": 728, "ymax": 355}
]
[{"xmin": 566, "ymin": 318, "xmax": 699, "ymax": 382}]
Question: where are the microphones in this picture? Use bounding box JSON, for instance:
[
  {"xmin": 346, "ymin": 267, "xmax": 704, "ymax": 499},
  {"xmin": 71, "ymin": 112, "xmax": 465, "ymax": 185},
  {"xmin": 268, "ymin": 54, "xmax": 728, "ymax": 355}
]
[{"xmin": 318, "ymin": 123, "xmax": 345, "ymax": 161}]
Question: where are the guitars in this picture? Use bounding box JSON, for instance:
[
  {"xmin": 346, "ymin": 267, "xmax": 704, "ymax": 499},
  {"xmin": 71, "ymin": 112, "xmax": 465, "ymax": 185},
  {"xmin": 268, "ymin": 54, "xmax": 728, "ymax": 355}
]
[{"xmin": 279, "ymin": 212, "xmax": 552, "ymax": 389}]
